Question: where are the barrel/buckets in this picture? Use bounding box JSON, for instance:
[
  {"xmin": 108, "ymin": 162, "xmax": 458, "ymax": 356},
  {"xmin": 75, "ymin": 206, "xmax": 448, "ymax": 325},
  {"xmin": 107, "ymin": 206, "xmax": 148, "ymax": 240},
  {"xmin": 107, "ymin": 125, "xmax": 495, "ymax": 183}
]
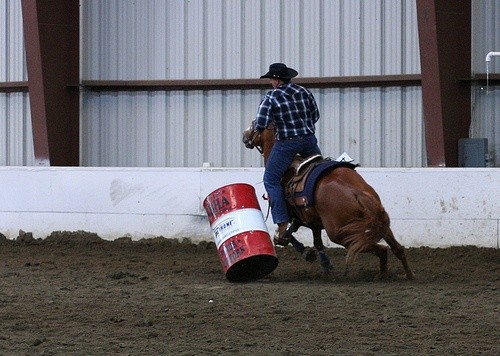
[{"xmin": 203, "ymin": 183, "xmax": 279, "ymax": 283}]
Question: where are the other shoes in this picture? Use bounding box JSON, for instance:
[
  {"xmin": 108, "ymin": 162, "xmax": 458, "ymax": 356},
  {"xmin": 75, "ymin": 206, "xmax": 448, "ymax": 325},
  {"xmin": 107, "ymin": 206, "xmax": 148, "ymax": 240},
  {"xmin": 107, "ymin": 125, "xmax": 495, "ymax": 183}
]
[{"xmin": 274, "ymin": 222, "xmax": 292, "ymax": 246}]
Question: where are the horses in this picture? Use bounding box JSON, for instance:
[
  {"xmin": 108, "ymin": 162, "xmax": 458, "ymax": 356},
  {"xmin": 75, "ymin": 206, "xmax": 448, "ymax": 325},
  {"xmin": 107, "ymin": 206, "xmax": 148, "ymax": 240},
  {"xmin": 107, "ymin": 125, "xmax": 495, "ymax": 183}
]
[{"xmin": 242, "ymin": 118, "xmax": 415, "ymax": 282}]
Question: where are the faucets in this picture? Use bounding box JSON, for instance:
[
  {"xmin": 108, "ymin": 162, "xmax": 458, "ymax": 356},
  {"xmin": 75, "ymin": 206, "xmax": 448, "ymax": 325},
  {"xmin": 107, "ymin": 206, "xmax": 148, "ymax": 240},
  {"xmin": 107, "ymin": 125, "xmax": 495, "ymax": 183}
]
[{"xmin": 486, "ymin": 51, "xmax": 500, "ymax": 62}]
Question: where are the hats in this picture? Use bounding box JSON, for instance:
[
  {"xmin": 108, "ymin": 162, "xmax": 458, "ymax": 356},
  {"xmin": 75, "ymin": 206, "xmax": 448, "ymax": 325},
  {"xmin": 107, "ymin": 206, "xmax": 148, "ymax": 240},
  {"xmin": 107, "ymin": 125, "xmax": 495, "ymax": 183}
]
[{"xmin": 260, "ymin": 63, "xmax": 298, "ymax": 79}]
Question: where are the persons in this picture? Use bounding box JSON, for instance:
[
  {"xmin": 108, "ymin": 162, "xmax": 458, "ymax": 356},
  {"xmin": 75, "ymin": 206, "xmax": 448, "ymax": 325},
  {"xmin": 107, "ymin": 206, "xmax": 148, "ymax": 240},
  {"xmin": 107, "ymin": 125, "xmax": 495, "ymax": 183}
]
[{"xmin": 251, "ymin": 62, "xmax": 322, "ymax": 245}]
